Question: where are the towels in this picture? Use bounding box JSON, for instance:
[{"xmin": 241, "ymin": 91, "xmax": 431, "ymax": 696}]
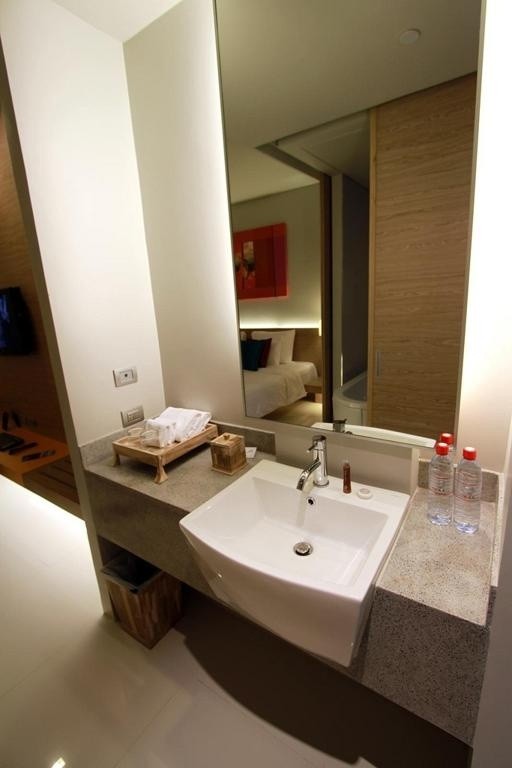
[{"xmin": 139, "ymin": 407, "xmax": 212, "ymax": 448}]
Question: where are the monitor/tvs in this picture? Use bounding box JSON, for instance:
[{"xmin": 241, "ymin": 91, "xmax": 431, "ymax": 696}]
[{"xmin": 0, "ymin": 285, "xmax": 42, "ymax": 357}]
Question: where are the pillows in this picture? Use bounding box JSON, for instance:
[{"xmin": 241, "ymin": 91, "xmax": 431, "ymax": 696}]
[{"xmin": 239, "ymin": 330, "xmax": 295, "ymax": 372}]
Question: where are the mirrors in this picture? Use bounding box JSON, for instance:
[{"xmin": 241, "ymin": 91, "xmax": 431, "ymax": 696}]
[{"xmin": 213, "ymin": 0, "xmax": 486, "ymax": 448}]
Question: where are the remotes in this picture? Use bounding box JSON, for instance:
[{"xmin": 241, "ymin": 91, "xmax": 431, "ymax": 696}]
[
  {"xmin": 8, "ymin": 442, "xmax": 38, "ymax": 454},
  {"xmin": 22, "ymin": 449, "xmax": 54, "ymax": 462}
]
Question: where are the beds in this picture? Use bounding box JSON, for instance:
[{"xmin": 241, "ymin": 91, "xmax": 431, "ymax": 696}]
[{"xmin": 239, "ymin": 328, "xmax": 319, "ymax": 417}]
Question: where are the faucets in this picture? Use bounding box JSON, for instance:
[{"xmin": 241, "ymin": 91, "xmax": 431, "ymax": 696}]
[{"xmin": 297, "ymin": 434, "xmax": 330, "ymax": 491}]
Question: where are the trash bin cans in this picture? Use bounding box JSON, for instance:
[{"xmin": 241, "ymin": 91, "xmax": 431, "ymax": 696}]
[{"xmin": 99, "ymin": 550, "xmax": 185, "ymax": 649}]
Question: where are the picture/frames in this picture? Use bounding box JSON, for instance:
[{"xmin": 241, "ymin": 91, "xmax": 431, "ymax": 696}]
[{"xmin": 234, "ymin": 224, "xmax": 287, "ymax": 299}]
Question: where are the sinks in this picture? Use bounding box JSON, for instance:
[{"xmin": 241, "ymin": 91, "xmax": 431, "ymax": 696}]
[
  {"xmin": 311, "ymin": 422, "xmax": 438, "ymax": 450},
  {"xmin": 179, "ymin": 459, "xmax": 410, "ymax": 667}
]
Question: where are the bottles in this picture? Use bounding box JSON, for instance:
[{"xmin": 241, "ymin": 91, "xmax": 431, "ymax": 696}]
[
  {"xmin": 454, "ymin": 447, "xmax": 481, "ymax": 534},
  {"xmin": 431, "ymin": 432, "xmax": 453, "ymax": 466},
  {"xmin": 427, "ymin": 443, "xmax": 453, "ymax": 525}
]
